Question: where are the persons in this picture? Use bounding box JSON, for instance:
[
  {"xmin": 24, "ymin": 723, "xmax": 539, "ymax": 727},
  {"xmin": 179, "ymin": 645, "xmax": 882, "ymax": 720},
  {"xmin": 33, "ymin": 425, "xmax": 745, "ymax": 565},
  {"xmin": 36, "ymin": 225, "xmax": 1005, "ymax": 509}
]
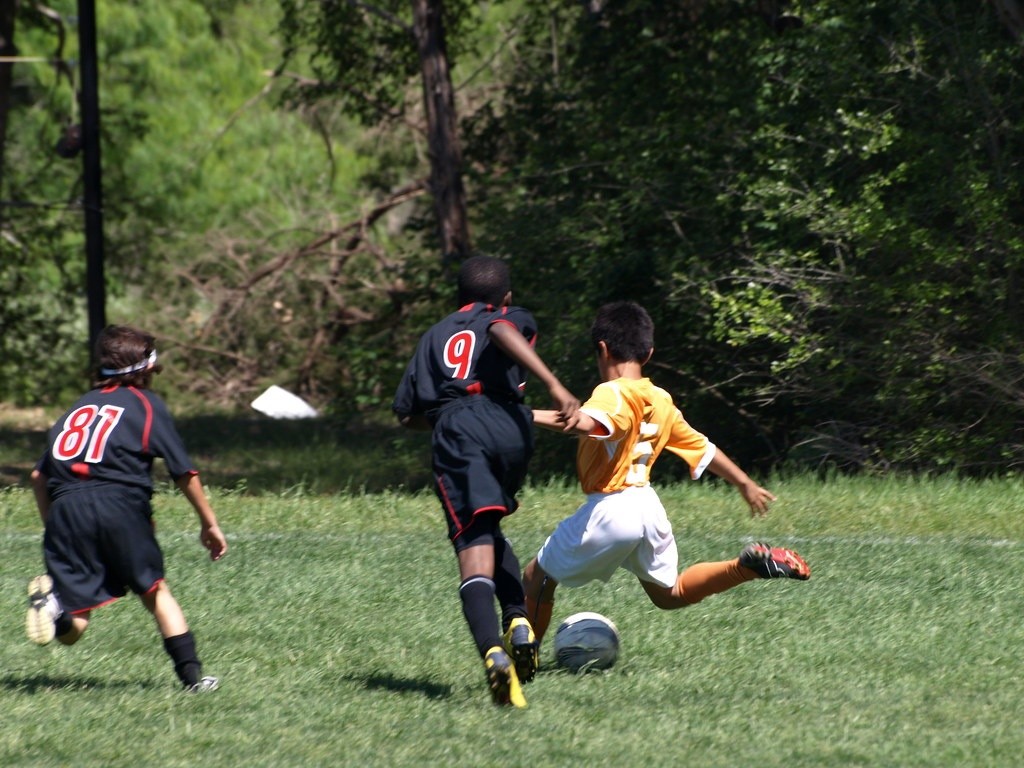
[
  {"xmin": 392, "ymin": 255, "xmax": 580, "ymax": 708},
  {"xmin": 521, "ymin": 301, "xmax": 811, "ymax": 656},
  {"xmin": 25, "ymin": 324, "xmax": 228, "ymax": 696}
]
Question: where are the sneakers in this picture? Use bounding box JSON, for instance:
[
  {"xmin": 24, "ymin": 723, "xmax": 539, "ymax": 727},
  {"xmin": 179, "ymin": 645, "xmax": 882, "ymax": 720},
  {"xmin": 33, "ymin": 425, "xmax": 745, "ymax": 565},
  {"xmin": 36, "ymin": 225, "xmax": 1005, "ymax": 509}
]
[
  {"xmin": 485, "ymin": 646, "xmax": 527, "ymax": 708},
  {"xmin": 739, "ymin": 541, "xmax": 810, "ymax": 580},
  {"xmin": 502, "ymin": 617, "xmax": 539, "ymax": 685}
]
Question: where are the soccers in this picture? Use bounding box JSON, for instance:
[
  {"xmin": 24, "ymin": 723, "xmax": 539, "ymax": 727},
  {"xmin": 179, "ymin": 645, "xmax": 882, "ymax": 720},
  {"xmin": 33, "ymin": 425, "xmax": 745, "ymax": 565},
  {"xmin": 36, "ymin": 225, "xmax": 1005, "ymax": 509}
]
[{"xmin": 552, "ymin": 610, "xmax": 623, "ymax": 677}]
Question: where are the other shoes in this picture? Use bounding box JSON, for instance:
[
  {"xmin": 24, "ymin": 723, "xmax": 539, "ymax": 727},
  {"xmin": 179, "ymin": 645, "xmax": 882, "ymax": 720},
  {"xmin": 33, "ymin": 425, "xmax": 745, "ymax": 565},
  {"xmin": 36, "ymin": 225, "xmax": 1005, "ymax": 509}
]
[
  {"xmin": 195, "ymin": 676, "xmax": 220, "ymax": 697},
  {"xmin": 25, "ymin": 574, "xmax": 60, "ymax": 645}
]
